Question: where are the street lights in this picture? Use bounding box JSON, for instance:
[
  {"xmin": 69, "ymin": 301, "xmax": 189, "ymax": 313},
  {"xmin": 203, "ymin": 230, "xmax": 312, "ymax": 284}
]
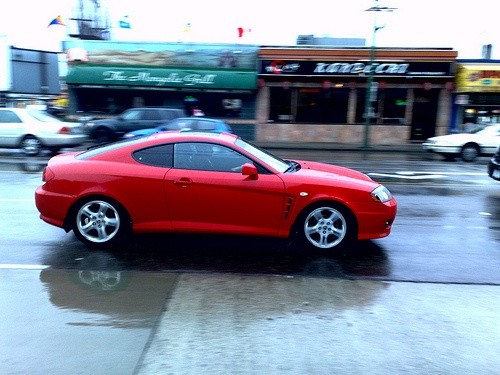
[{"xmin": 361, "ymin": 7, "xmax": 388, "ymax": 149}]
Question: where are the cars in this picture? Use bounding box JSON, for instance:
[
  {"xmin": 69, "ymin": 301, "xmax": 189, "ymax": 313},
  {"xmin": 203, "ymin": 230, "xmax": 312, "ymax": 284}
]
[
  {"xmin": 34, "ymin": 130, "xmax": 398, "ymax": 256},
  {"xmin": 422, "ymin": 122, "xmax": 500, "ymax": 163},
  {"xmin": 122, "ymin": 117, "xmax": 232, "ymax": 145},
  {"xmin": 0, "ymin": 106, "xmax": 90, "ymax": 156},
  {"xmin": 84, "ymin": 107, "xmax": 186, "ymax": 144}
]
